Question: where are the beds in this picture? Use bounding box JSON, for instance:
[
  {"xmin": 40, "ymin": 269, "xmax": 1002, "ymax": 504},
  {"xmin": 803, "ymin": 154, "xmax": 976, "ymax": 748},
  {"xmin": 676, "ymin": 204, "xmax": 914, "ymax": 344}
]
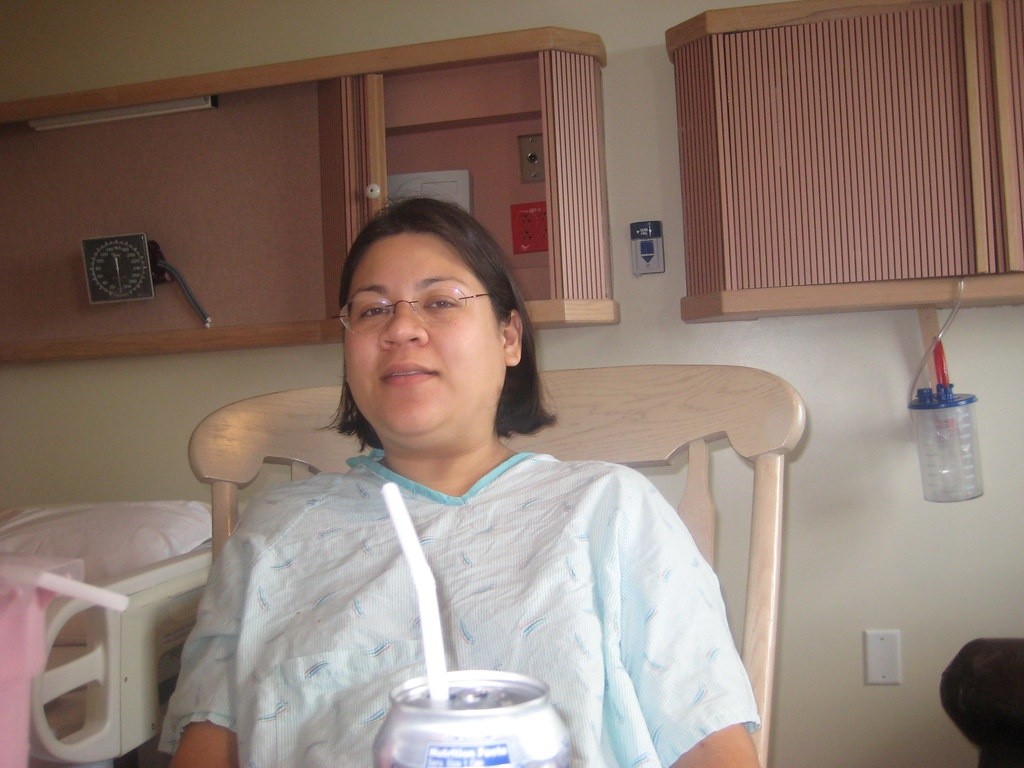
[{"xmin": 0, "ymin": 548, "xmax": 216, "ymax": 768}]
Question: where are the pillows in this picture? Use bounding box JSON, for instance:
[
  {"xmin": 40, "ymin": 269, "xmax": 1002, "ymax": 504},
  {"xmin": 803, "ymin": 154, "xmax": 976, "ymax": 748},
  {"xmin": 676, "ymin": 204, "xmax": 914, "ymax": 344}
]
[{"xmin": 1, "ymin": 499, "xmax": 213, "ymax": 644}]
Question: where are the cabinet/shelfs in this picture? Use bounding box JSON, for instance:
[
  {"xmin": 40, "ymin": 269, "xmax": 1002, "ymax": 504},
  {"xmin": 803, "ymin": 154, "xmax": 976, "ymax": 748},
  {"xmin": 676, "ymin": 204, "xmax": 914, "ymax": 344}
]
[
  {"xmin": 0, "ymin": 27, "xmax": 622, "ymax": 364},
  {"xmin": 664, "ymin": 1, "xmax": 1024, "ymax": 323}
]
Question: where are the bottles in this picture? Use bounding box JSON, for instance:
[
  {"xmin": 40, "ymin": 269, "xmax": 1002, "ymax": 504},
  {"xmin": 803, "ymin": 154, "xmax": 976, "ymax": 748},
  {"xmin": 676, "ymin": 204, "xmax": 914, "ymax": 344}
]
[{"xmin": 909, "ymin": 385, "xmax": 984, "ymax": 503}]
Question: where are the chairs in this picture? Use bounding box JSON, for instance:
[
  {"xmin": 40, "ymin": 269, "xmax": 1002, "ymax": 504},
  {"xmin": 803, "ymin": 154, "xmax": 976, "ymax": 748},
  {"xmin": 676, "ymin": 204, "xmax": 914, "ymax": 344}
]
[{"xmin": 186, "ymin": 364, "xmax": 807, "ymax": 768}]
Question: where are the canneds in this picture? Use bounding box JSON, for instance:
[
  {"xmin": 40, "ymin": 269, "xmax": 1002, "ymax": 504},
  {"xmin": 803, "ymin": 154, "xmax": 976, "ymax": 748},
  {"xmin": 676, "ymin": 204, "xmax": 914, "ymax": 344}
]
[{"xmin": 372, "ymin": 669, "xmax": 573, "ymax": 768}]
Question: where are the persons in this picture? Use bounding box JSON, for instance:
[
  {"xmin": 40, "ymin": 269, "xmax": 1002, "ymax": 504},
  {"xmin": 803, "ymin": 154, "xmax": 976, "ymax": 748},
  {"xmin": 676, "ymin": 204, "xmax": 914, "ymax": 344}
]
[{"xmin": 158, "ymin": 196, "xmax": 762, "ymax": 768}]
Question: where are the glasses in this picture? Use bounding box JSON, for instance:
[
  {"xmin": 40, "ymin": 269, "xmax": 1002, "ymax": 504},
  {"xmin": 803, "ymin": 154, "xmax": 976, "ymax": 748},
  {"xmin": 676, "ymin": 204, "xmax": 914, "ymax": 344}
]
[{"xmin": 332, "ymin": 288, "xmax": 491, "ymax": 334}]
